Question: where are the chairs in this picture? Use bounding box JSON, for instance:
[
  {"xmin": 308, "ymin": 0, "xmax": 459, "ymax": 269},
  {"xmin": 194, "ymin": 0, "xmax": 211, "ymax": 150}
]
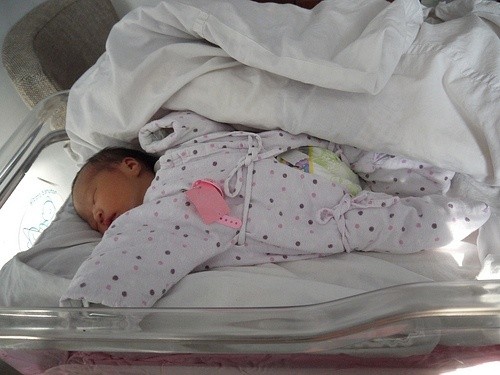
[{"xmin": 1, "ymin": 0, "xmax": 119, "ymax": 132}]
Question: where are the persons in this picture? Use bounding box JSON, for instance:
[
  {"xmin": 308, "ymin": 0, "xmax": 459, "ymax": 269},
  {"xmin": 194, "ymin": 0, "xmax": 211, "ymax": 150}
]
[{"xmin": 58, "ymin": 110, "xmax": 491, "ymax": 323}]
